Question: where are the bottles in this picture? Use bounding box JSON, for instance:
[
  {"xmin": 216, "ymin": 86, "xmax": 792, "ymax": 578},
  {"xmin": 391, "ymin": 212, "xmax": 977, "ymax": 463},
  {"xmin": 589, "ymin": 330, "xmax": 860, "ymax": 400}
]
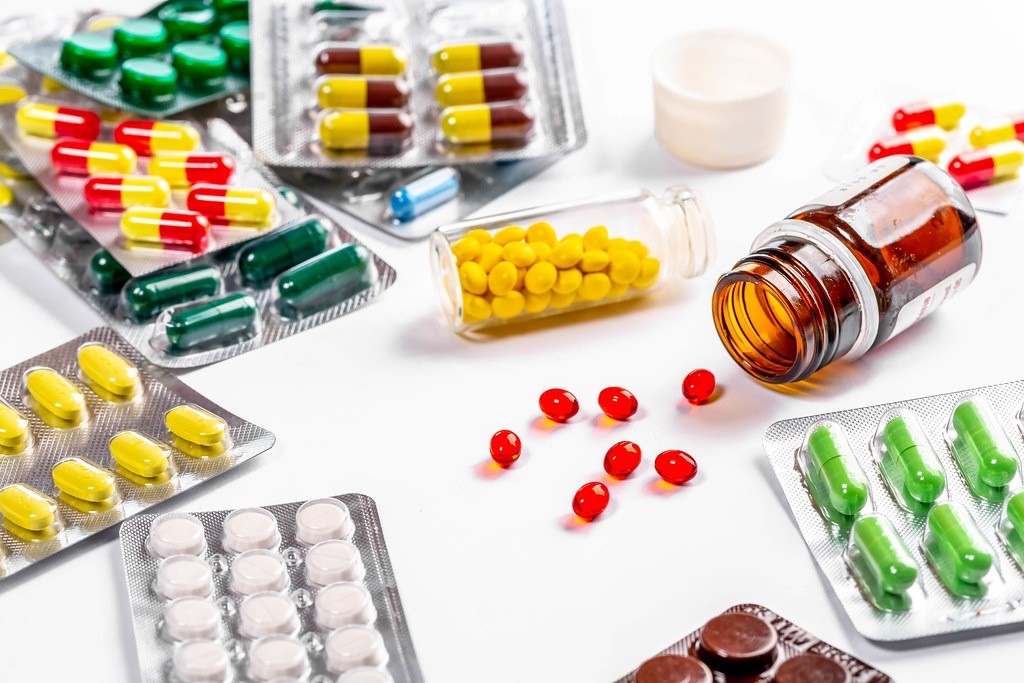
[{"xmin": 712, "ymin": 155, "xmax": 983, "ymax": 382}]
